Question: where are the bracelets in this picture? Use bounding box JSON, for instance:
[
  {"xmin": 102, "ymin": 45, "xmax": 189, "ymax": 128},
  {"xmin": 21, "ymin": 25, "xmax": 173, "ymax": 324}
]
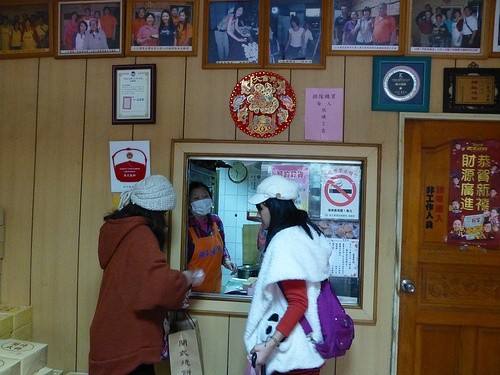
[{"xmin": 270, "ymin": 336, "xmax": 279, "ymax": 346}]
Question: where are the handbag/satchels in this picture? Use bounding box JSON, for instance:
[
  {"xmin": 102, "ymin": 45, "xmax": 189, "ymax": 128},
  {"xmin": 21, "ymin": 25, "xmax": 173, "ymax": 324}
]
[{"xmin": 167, "ymin": 308, "xmax": 204, "ymax": 375}]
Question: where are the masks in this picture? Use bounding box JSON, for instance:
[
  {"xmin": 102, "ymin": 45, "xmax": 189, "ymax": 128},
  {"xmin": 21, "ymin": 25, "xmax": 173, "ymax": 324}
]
[{"xmin": 190, "ymin": 199, "xmax": 213, "ymax": 216}]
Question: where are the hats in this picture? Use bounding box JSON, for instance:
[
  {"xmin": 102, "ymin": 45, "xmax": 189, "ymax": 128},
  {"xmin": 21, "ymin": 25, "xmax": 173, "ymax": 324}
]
[
  {"xmin": 248, "ymin": 174, "xmax": 299, "ymax": 205},
  {"xmin": 117, "ymin": 174, "xmax": 177, "ymax": 212}
]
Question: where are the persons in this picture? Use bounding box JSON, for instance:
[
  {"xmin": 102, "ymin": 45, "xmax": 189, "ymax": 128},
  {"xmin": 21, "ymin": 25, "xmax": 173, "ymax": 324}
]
[
  {"xmin": 415, "ymin": 3, "xmax": 478, "ymax": 47},
  {"xmin": 132, "ymin": 6, "xmax": 193, "ymax": 47},
  {"xmin": 214, "ymin": 3, "xmax": 250, "ymax": 61},
  {"xmin": 89, "ymin": 175, "xmax": 206, "ymax": 375},
  {"xmin": 186, "ymin": 180, "xmax": 238, "ymax": 293},
  {"xmin": 269, "ymin": 25, "xmax": 280, "ymax": 64},
  {"xmin": 0, "ymin": 13, "xmax": 49, "ymax": 50},
  {"xmin": 334, "ymin": 2, "xmax": 396, "ymax": 46},
  {"xmin": 244, "ymin": 176, "xmax": 334, "ymax": 375},
  {"xmin": 283, "ymin": 17, "xmax": 305, "ymax": 60},
  {"xmin": 63, "ymin": 6, "xmax": 118, "ymax": 50}
]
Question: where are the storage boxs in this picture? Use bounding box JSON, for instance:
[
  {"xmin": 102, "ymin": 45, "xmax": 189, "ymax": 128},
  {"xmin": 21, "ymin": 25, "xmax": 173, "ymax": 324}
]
[{"xmin": 0, "ymin": 304, "xmax": 48, "ymax": 375}]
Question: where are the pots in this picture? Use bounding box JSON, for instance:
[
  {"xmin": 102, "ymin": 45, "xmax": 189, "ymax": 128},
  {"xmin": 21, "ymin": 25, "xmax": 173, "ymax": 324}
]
[{"xmin": 238, "ymin": 265, "xmax": 259, "ymax": 279}]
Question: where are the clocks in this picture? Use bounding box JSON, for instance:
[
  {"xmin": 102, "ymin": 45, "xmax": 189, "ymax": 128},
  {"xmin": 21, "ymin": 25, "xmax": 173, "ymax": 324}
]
[{"xmin": 228, "ymin": 161, "xmax": 247, "ymax": 183}]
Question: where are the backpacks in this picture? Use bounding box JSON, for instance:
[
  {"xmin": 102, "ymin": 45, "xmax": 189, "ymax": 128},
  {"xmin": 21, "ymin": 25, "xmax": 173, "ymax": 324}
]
[{"xmin": 298, "ymin": 280, "xmax": 355, "ymax": 360}]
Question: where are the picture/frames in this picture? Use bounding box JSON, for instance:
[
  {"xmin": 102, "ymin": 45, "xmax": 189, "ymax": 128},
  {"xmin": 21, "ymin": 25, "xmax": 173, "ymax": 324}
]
[{"xmin": 0, "ymin": 0, "xmax": 500, "ymax": 70}]
